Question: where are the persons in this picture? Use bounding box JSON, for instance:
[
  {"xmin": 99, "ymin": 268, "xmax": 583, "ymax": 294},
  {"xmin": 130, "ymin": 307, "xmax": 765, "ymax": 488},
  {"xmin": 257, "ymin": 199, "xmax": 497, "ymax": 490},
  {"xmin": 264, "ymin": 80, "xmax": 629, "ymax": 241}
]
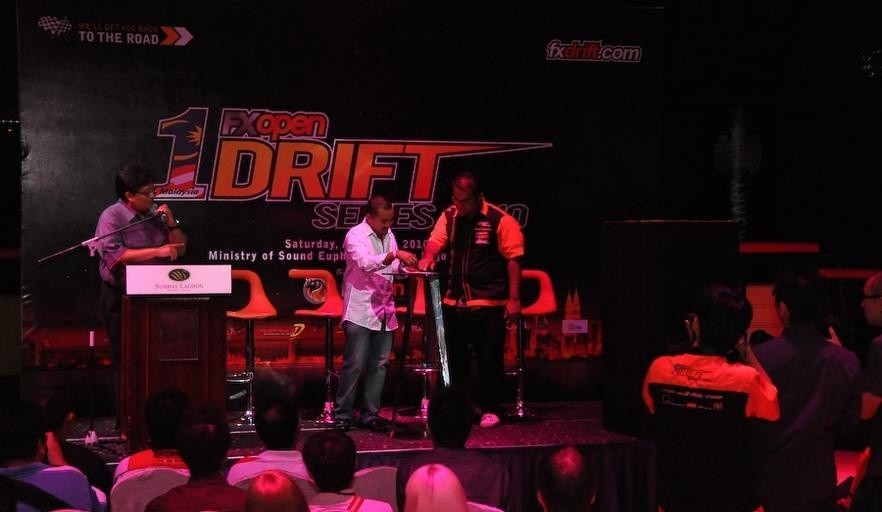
[
  {"xmin": 94, "ymin": 161, "xmax": 187, "ymax": 436},
  {"xmin": 334, "ymin": 196, "xmax": 418, "ymax": 433},
  {"xmin": 2, "ymin": 264, "xmax": 879, "ymax": 512},
  {"xmin": 420, "ymin": 172, "xmax": 526, "ymax": 429}
]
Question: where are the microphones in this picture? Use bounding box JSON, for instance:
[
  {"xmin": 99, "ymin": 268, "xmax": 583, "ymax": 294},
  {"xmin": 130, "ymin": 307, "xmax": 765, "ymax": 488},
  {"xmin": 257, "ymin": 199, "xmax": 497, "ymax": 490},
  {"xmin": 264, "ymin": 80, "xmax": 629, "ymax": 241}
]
[{"xmin": 150, "ymin": 204, "xmax": 167, "ymax": 223}]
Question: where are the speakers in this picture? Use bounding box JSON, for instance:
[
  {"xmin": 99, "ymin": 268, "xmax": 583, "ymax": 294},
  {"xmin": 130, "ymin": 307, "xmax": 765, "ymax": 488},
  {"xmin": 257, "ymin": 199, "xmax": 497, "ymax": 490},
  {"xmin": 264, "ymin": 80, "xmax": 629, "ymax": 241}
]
[{"xmin": 602, "ymin": 217, "xmax": 746, "ymax": 442}]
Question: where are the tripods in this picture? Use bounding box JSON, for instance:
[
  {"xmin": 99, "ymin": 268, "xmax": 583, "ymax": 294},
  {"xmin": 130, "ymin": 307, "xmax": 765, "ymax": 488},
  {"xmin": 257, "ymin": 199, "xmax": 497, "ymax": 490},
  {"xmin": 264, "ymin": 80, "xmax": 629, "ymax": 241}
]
[{"xmin": 66, "ymin": 268, "xmax": 120, "ymax": 458}]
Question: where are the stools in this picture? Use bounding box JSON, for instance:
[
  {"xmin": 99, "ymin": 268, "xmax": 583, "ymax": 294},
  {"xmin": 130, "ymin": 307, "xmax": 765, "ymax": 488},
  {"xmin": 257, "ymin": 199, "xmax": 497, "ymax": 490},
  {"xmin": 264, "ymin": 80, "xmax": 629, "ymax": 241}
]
[
  {"xmin": 395, "ymin": 269, "xmax": 558, "ymax": 428},
  {"xmin": 226, "ymin": 269, "xmax": 278, "ymax": 434},
  {"xmin": 288, "ymin": 269, "xmax": 346, "ymax": 433}
]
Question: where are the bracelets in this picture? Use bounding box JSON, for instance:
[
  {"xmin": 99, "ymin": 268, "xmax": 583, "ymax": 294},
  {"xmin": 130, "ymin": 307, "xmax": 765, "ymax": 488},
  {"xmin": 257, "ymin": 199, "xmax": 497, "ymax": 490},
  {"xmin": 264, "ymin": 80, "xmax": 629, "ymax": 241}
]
[
  {"xmin": 167, "ymin": 219, "xmax": 180, "ymax": 230},
  {"xmin": 392, "ymin": 249, "xmax": 398, "ymax": 258}
]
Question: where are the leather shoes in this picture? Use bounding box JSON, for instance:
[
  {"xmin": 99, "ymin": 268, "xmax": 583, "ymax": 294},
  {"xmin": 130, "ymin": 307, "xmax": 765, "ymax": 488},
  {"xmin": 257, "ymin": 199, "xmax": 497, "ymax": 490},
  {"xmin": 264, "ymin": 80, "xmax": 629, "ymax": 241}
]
[
  {"xmin": 359, "ymin": 419, "xmax": 393, "ymax": 430},
  {"xmin": 336, "ymin": 418, "xmax": 350, "ymax": 431}
]
